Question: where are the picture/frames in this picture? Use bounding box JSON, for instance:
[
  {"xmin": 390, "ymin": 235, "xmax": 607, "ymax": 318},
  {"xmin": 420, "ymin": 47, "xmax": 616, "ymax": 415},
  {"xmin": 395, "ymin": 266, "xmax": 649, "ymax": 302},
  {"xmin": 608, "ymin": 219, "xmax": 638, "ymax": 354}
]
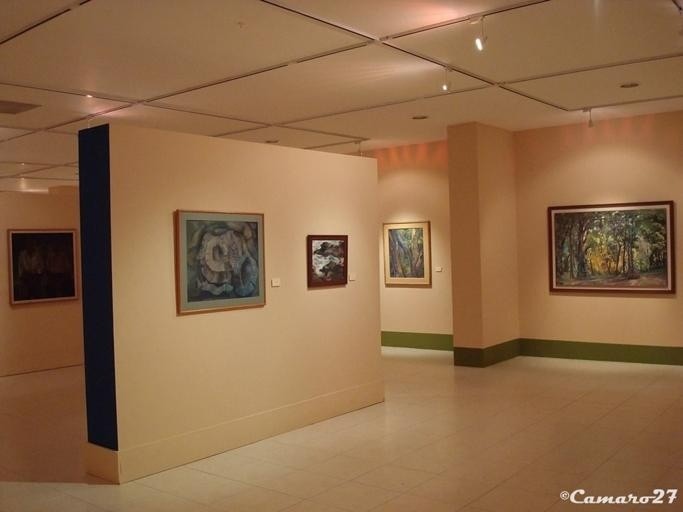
[
  {"xmin": 547, "ymin": 201, "xmax": 676, "ymax": 295},
  {"xmin": 173, "ymin": 199, "xmax": 432, "ymax": 319},
  {"xmin": 5, "ymin": 226, "xmax": 80, "ymax": 307}
]
